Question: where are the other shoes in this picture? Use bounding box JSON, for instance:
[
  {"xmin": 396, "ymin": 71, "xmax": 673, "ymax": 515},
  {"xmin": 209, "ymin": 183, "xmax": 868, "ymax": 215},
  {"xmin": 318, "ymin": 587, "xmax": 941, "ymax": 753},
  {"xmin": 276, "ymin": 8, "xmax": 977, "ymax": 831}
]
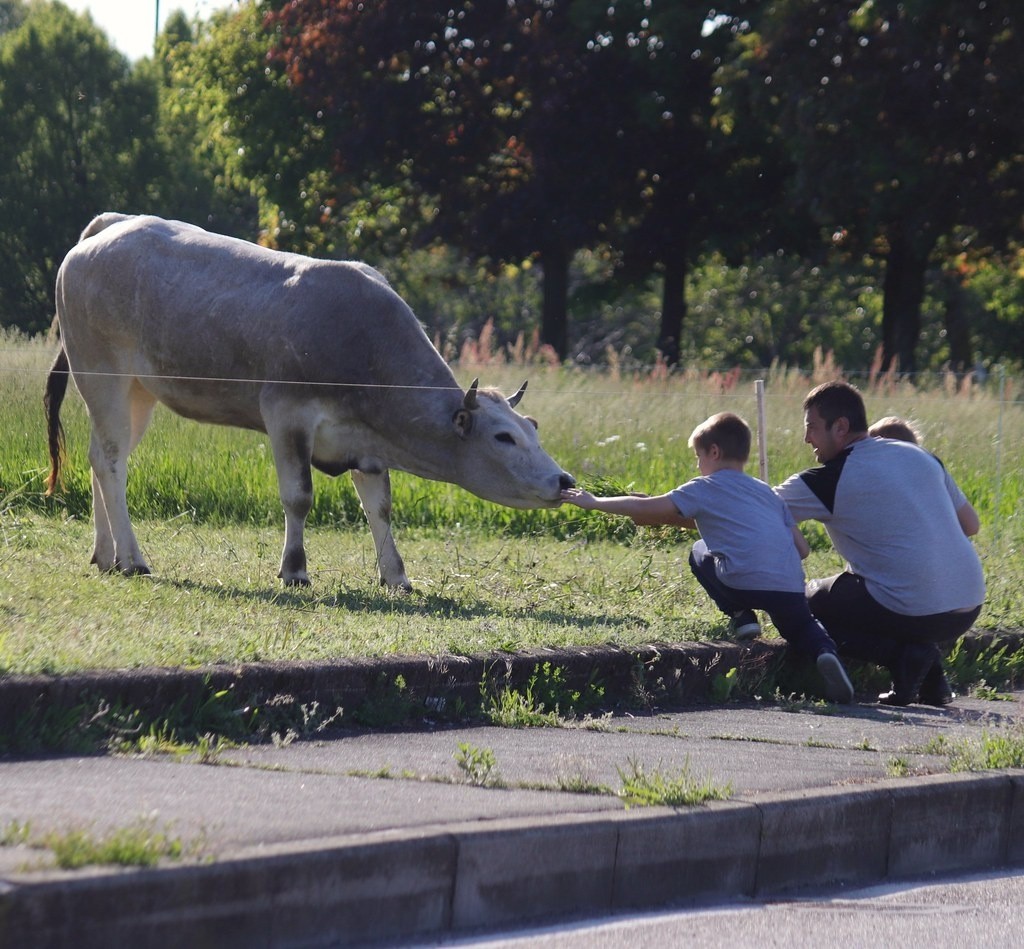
[
  {"xmin": 914, "ymin": 685, "xmax": 951, "ymax": 705},
  {"xmin": 729, "ymin": 609, "xmax": 762, "ymax": 641},
  {"xmin": 878, "ymin": 642, "xmax": 937, "ymax": 705},
  {"xmin": 817, "ymin": 647, "xmax": 853, "ymax": 704}
]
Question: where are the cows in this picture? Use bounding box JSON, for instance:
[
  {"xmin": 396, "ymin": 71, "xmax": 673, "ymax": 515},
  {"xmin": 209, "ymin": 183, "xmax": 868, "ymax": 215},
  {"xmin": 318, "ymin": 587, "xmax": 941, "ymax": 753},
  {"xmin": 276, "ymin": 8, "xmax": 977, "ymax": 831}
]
[{"xmin": 40, "ymin": 211, "xmax": 578, "ymax": 592}]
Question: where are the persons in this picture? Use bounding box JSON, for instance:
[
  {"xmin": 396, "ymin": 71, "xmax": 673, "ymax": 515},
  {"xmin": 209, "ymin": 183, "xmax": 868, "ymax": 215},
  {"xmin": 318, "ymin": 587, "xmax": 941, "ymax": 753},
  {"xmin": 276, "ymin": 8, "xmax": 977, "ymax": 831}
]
[
  {"xmin": 560, "ymin": 412, "xmax": 854, "ymax": 703},
  {"xmin": 628, "ymin": 380, "xmax": 986, "ymax": 706}
]
